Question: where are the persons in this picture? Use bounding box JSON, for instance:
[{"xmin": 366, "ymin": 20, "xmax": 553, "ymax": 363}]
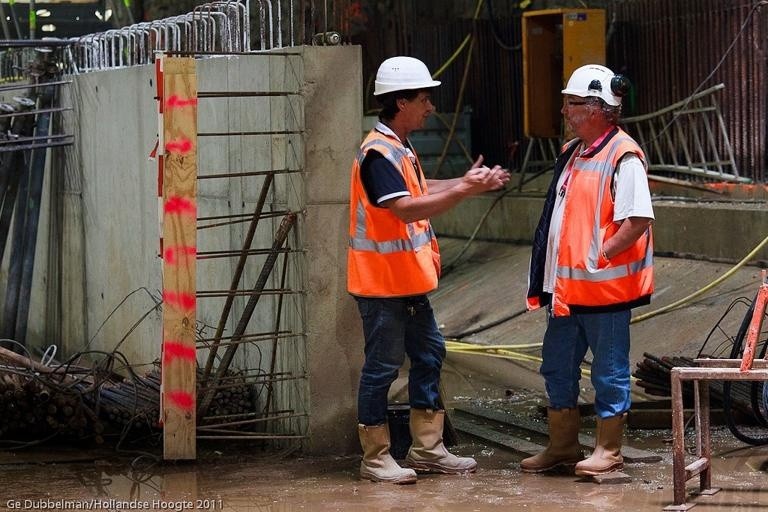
[
  {"xmin": 519, "ymin": 65, "xmax": 654, "ymax": 477},
  {"xmin": 346, "ymin": 55, "xmax": 511, "ymax": 484}
]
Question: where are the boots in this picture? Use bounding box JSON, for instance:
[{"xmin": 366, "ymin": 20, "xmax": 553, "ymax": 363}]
[
  {"xmin": 575, "ymin": 414, "xmax": 628, "ymax": 476},
  {"xmin": 520, "ymin": 407, "xmax": 583, "ymax": 473},
  {"xmin": 358, "ymin": 422, "xmax": 419, "ymax": 485},
  {"xmin": 403, "ymin": 408, "xmax": 477, "ymax": 476}
]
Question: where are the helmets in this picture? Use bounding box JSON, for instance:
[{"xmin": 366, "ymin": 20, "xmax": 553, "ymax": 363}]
[
  {"xmin": 560, "ymin": 64, "xmax": 632, "ymax": 107},
  {"xmin": 372, "ymin": 55, "xmax": 443, "ymax": 96}
]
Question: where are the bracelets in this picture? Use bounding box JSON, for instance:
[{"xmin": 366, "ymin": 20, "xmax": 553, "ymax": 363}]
[{"xmin": 601, "ymin": 249, "xmax": 608, "ymax": 261}]
